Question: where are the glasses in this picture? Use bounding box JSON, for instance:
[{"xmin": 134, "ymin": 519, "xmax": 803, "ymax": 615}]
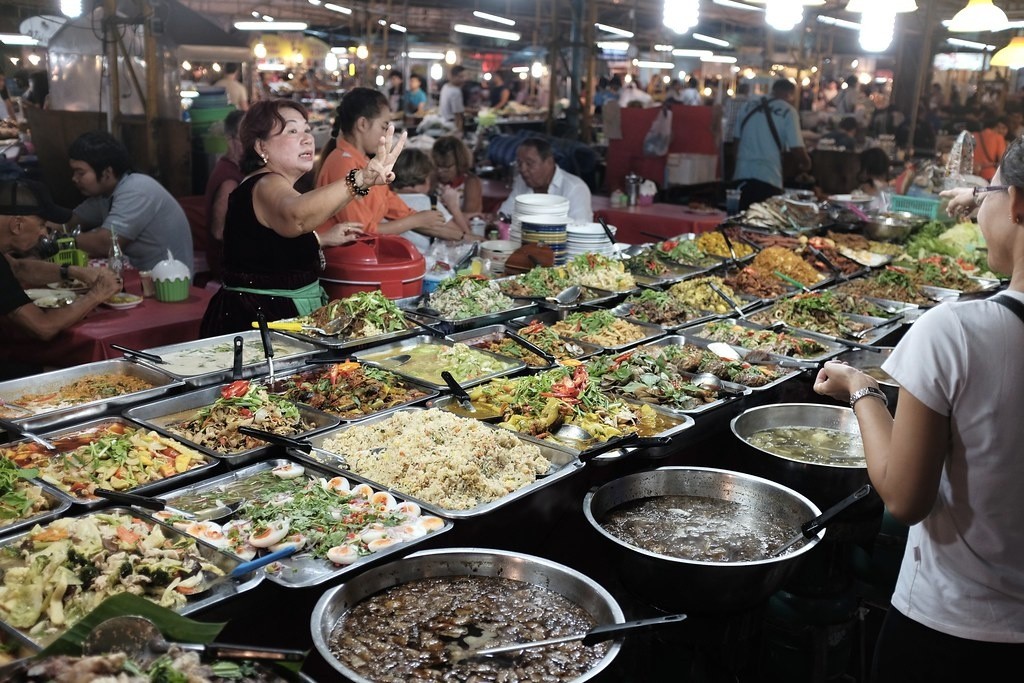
[{"xmin": 973, "ymin": 186, "xmax": 1007, "ymax": 207}]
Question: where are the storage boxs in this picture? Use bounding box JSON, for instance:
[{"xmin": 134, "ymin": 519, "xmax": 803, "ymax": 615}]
[{"xmin": 664, "ymin": 153, "xmax": 717, "ymax": 186}]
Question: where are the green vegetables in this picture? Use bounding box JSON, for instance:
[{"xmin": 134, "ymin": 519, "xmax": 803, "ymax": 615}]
[
  {"xmin": 237, "ymin": 473, "xmax": 405, "ymax": 561},
  {"xmin": 484, "ymin": 240, "xmax": 850, "ymax": 417},
  {"xmin": 867, "ymin": 220, "xmax": 1010, "ymax": 318},
  {"xmin": 438, "ymin": 274, "xmax": 506, "ymax": 319},
  {"xmin": 98, "ymin": 653, "xmax": 260, "ymax": 683},
  {"xmin": 198, "ymin": 339, "xmax": 397, "ymax": 425},
  {"xmin": 328, "ymin": 290, "xmax": 409, "ymax": 331},
  {"xmin": 0, "ymin": 427, "xmax": 201, "ymax": 634}
]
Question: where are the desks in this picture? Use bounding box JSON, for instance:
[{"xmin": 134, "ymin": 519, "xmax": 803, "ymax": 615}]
[
  {"xmin": 42, "ymin": 287, "xmax": 212, "ymax": 368},
  {"xmin": 479, "ymin": 176, "xmax": 726, "ymax": 255}
]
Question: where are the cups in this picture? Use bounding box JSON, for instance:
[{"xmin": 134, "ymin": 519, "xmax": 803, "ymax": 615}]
[
  {"xmin": 470, "ymin": 217, "xmax": 485, "ymax": 237},
  {"xmin": 725, "ymin": 189, "xmax": 742, "ymax": 215}
]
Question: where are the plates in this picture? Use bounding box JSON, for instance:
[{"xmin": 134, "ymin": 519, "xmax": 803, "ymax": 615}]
[
  {"xmin": 46, "ymin": 279, "xmax": 91, "ymax": 293},
  {"xmin": 479, "ymin": 193, "xmax": 617, "ymax": 277},
  {"xmin": 33, "ymin": 294, "xmax": 87, "ymax": 312},
  {"xmin": 23, "ymin": 289, "xmax": 76, "ymax": 299},
  {"xmin": 684, "ymin": 208, "xmax": 721, "ymax": 214},
  {"xmin": 102, "ymin": 292, "xmax": 142, "ymax": 309}
]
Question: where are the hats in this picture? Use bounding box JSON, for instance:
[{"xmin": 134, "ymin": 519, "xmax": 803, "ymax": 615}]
[{"xmin": 0, "ymin": 174, "xmax": 72, "ymax": 223}]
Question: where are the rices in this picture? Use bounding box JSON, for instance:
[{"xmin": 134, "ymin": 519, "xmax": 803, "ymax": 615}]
[{"xmin": 322, "ymin": 407, "xmax": 552, "ymax": 510}]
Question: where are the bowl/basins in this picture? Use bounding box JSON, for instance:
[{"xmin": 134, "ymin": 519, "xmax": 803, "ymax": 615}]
[
  {"xmin": 310, "ymin": 548, "xmax": 626, "ymax": 683},
  {"xmin": 0, "ymin": 654, "xmax": 317, "ymax": 683},
  {"xmin": 832, "ymin": 346, "xmax": 918, "ymax": 406},
  {"xmin": 827, "ymin": 195, "xmax": 929, "ymax": 245},
  {"xmin": 730, "ymin": 402, "xmax": 894, "ymax": 505},
  {"xmin": 196, "ymin": 85, "xmax": 226, "ymax": 94},
  {"xmin": 583, "ymin": 466, "xmax": 825, "ymax": 603},
  {"xmin": 788, "ymin": 187, "xmax": 815, "ymax": 200}
]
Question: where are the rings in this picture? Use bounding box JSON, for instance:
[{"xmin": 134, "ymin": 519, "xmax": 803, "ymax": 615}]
[{"xmin": 117, "ymin": 278, "xmax": 120, "ymax": 283}]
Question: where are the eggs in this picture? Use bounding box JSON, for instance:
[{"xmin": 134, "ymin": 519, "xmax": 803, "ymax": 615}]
[
  {"xmin": 154, "ymin": 520, "xmax": 305, "ymax": 564},
  {"xmin": 272, "ymin": 466, "xmax": 446, "ymax": 565}
]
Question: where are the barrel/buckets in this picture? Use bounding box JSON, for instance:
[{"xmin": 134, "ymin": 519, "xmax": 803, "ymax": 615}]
[
  {"xmin": 189, "ymin": 96, "xmax": 237, "ymax": 122},
  {"xmin": 423, "ymin": 261, "xmax": 455, "ymax": 294},
  {"xmin": 200, "ymin": 134, "xmax": 228, "ymax": 153}
]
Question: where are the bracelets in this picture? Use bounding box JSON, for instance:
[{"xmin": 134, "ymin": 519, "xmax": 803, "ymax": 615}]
[
  {"xmin": 61, "ymin": 263, "xmax": 74, "ymax": 281},
  {"xmin": 346, "ymin": 173, "xmax": 358, "ymax": 199},
  {"xmin": 350, "ymin": 169, "xmax": 369, "ymax": 196},
  {"xmin": 461, "ymin": 232, "xmax": 465, "ymax": 240},
  {"xmin": 850, "ymin": 387, "xmax": 888, "ymax": 414}
]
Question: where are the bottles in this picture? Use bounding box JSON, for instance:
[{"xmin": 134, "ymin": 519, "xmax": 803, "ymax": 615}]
[
  {"xmin": 109, "ymin": 237, "xmax": 126, "ymax": 293},
  {"xmin": 489, "ymin": 230, "xmax": 498, "ymax": 240},
  {"xmin": 625, "ymin": 170, "xmax": 643, "ymax": 205}
]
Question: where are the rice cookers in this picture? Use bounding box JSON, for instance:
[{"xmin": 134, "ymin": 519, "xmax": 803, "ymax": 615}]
[{"xmin": 318, "ymin": 234, "xmax": 426, "ymax": 301}]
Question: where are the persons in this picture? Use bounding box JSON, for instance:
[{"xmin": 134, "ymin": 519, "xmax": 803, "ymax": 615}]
[
  {"xmin": 493, "ymin": 138, "xmax": 594, "ymax": 223},
  {"xmin": 432, "ymin": 137, "xmax": 483, "ymax": 214},
  {"xmin": 806, "ymin": 76, "xmax": 892, "ymax": 177},
  {"xmin": 388, "ymin": 71, "xmax": 427, "ymax": 113},
  {"xmin": 0, "ymin": 69, "xmax": 31, "ymax": 180},
  {"xmin": 0, "ymin": 177, "xmax": 123, "ymax": 375},
  {"xmin": 214, "ymin": 63, "xmax": 250, "ymax": 111},
  {"xmin": 389, "ymin": 148, "xmax": 473, "ymax": 250},
  {"xmin": 592, "ymin": 73, "xmax": 749, "ymax": 182},
  {"xmin": 732, "ymin": 80, "xmax": 810, "ymax": 208},
  {"xmin": 205, "ymin": 110, "xmax": 247, "ymax": 276},
  {"xmin": 921, "ymin": 83, "xmax": 1024, "ymax": 184},
  {"xmin": 813, "ymin": 140, "xmax": 1023, "ymax": 683},
  {"xmin": 199, "ymin": 98, "xmax": 408, "ymax": 342},
  {"xmin": 439, "ymin": 66, "xmax": 465, "ymax": 130},
  {"xmin": 183, "ymin": 62, "xmax": 204, "ymax": 81},
  {"xmin": 482, "ymin": 73, "xmax": 512, "ymax": 110},
  {"xmin": 316, "ymin": 86, "xmax": 487, "ymax": 243},
  {"xmin": 48, "ymin": 131, "xmax": 194, "ymax": 286}
]
